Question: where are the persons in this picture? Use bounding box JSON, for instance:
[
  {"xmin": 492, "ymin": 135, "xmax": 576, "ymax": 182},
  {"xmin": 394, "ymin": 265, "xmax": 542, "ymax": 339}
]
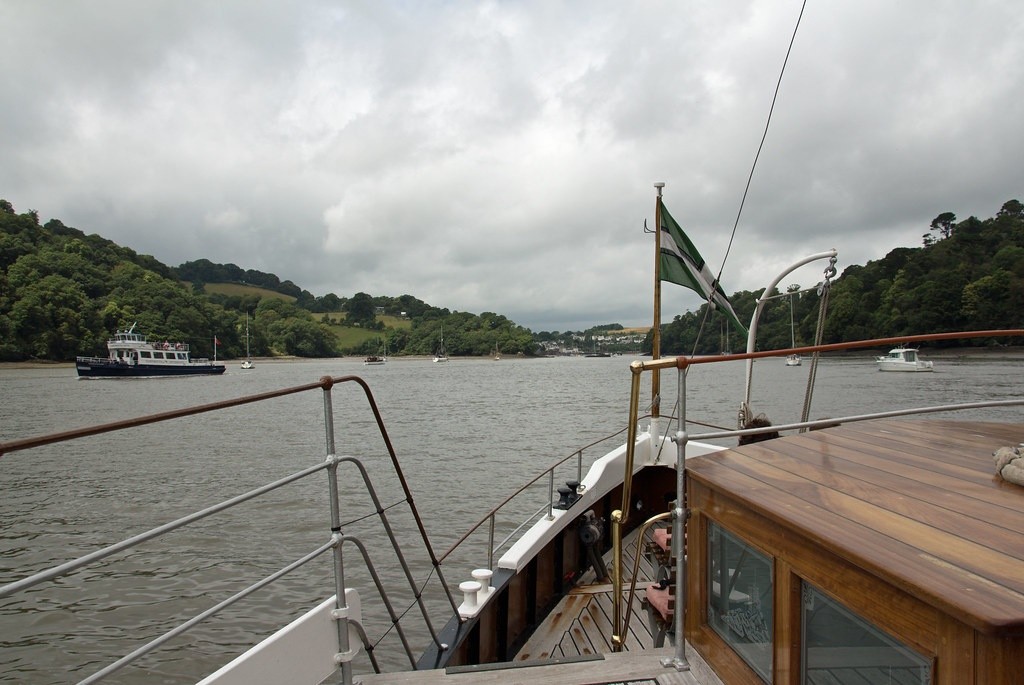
[
  {"xmin": 809, "ymin": 417, "xmax": 841, "ymax": 432},
  {"xmin": 157, "ymin": 340, "xmax": 181, "ymax": 350},
  {"xmin": 119, "ymin": 357, "xmax": 124, "ymax": 362},
  {"xmin": 738, "ymin": 413, "xmax": 779, "ymax": 447},
  {"xmin": 133, "ymin": 354, "xmax": 138, "ymax": 365}
]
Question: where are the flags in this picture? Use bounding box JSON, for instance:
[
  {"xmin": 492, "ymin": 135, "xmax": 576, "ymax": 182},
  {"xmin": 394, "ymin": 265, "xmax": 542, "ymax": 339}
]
[
  {"xmin": 215, "ymin": 338, "xmax": 222, "ymax": 345},
  {"xmin": 659, "ymin": 199, "xmax": 748, "ymax": 338}
]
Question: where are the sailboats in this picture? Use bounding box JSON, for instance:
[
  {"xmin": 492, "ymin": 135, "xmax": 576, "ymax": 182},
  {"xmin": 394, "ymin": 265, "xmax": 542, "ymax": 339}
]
[
  {"xmin": 783, "ymin": 294, "xmax": 803, "ymax": 366},
  {"xmin": 432, "ymin": 324, "xmax": 450, "ymax": 363}
]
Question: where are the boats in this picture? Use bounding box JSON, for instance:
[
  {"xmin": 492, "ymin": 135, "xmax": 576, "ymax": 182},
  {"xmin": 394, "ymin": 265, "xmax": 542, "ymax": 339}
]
[
  {"xmin": 873, "ymin": 346, "xmax": 934, "ymax": 372},
  {"xmin": 362, "ymin": 354, "xmax": 387, "ymax": 366},
  {"xmin": 242, "ymin": 359, "xmax": 255, "ymax": 369},
  {"xmin": 75, "ymin": 320, "xmax": 226, "ymax": 377}
]
